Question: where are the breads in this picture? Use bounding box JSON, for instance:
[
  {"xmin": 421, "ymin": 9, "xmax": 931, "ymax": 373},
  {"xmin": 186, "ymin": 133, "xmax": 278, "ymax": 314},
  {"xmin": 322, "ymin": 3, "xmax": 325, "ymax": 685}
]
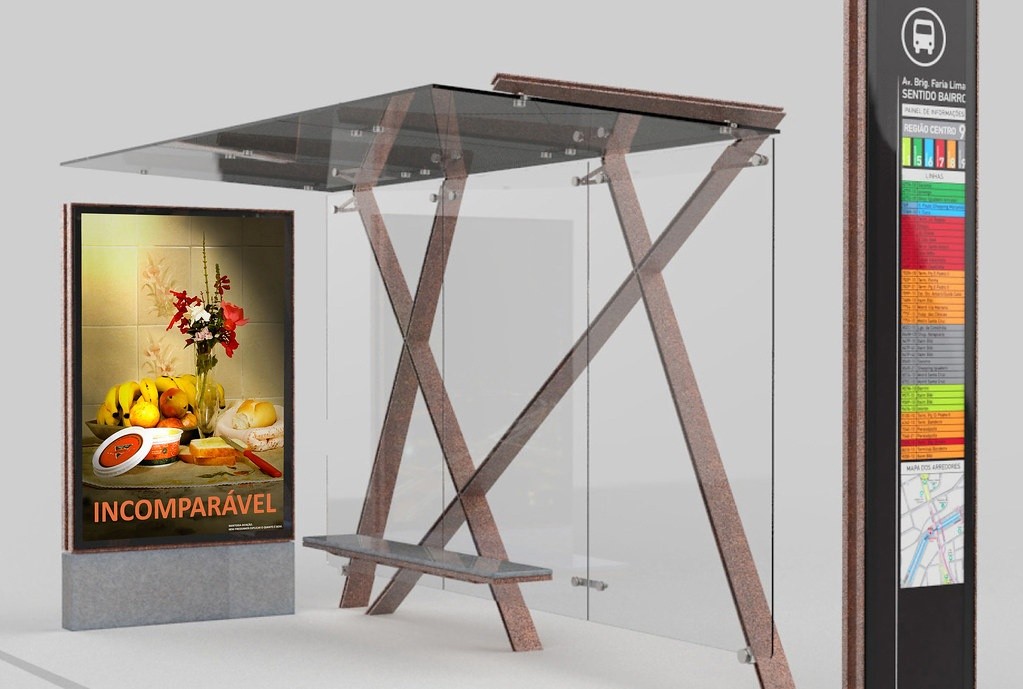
[
  {"xmin": 178, "ymin": 436, "xmax": 249, "ymax": 466},
  {"xmin": 237, "ymin": 399, "xmax": 277, "ymax": 428}
]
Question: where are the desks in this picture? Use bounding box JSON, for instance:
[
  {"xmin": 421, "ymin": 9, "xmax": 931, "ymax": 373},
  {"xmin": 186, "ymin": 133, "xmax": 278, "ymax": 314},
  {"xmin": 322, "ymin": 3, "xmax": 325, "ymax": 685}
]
[{"xmin": 81, "ymin": 436, "xmax": 283, "ymax": 540}]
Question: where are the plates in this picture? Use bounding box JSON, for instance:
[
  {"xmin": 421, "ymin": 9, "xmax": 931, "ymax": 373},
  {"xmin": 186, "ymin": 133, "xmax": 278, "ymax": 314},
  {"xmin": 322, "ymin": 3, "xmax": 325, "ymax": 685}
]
[{"xmin": 85, "ymin": 419, "xmax": 213, "ymax": 446}]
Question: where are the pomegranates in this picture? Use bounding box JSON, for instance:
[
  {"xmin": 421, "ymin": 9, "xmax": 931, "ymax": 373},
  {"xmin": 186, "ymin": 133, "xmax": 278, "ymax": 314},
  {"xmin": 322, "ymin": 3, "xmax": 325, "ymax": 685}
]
[
  {"xmin": 181, "ymin": 413, "xmax": 198, "ymax": 429},
  {"xmin": 156, "ymin": 417, "xmax": 183, "ymax": 428},
  {"xmin": 159, "ymin": 387, "xmax": 189, "ymax": 417}
]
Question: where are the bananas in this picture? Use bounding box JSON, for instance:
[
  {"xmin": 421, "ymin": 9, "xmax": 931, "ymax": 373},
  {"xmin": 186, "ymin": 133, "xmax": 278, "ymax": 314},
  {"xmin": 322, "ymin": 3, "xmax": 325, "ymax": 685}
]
[{"xmin": 96, "ymin": 374, "xmax": 227, "ymax": 426}]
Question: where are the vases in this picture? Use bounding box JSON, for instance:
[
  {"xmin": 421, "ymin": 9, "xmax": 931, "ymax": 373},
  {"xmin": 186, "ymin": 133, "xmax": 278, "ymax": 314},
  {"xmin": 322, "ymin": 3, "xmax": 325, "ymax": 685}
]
[{"xmin": 194, "ymin": 341, "xmax": 219, "ymax": 439}]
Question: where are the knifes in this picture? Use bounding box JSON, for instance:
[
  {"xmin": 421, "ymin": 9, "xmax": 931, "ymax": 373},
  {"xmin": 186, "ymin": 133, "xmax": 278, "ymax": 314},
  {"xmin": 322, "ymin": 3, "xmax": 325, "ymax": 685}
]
[{"xmin": 219, "ymin": 435, "xmax": 282, "ymax": 478}]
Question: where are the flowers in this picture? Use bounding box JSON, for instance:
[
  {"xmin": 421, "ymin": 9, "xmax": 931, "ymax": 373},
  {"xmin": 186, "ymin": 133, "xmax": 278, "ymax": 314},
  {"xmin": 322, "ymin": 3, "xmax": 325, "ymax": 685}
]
[{"xmin": 166, "ymin": 230, "xmax": 251, "ymax": 408}]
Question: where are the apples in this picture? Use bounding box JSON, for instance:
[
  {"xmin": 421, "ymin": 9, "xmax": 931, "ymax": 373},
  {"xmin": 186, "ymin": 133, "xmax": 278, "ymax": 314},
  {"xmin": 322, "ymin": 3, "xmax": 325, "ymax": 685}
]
[{"xmin": 130, "ymin": 401, "xmax": 160, "ymax": 427}]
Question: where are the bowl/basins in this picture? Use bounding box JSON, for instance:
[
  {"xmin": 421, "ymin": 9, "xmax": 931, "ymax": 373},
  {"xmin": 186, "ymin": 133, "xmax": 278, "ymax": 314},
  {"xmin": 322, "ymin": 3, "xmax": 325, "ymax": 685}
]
[{"xmin": 136, "ymin": 428, "xmax": 184, "ymax": 468}]
